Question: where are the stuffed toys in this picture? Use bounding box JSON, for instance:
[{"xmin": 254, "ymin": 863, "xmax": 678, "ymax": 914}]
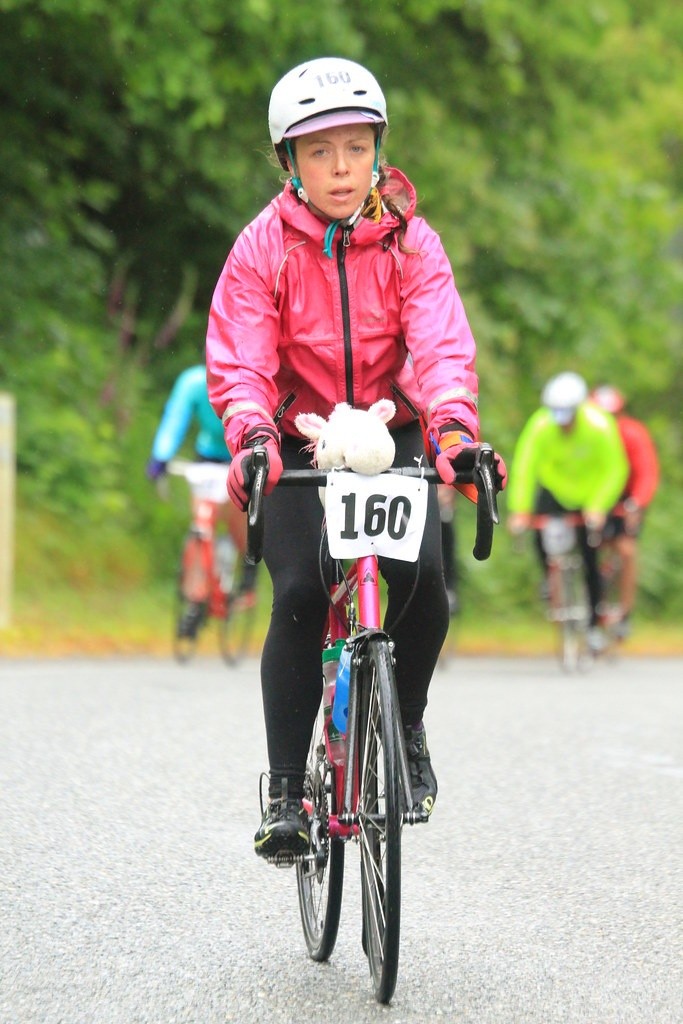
[{"xmin": 294, "ymin": 398, "xmax": 396, "ymax": 505}]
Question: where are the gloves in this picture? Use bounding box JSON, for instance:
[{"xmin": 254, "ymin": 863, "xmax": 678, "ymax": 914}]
[
  {"xmin": 436, "ymin": 430, "xmax": 508, "ymax": 492},
  {"xmin": 225, "ymin": 423, "xmax": 285, "ymax": 512},
  {"xmin": 143, "ymin": 457, "xmax": 167, "ymax": 480}
]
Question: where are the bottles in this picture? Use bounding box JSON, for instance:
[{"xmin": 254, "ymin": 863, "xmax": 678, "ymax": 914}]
[
  {"xmin": 321, "ymin": 639, "xmax": 348, "ymax": 767},
  {"xmin": 331, "ymin": 644, "xmax": 354, "ymax": 734}
]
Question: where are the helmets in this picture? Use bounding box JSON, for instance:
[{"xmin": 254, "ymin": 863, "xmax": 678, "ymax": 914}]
[
  {"xmin": 591, "ymin": 385, "xmax": 624, "ymax": 413},
  {"xmin": 267, "ymin": 57, "xmax": 389, "ymax": 172},
  {"xmin": 540, "ymin": 370, "xmax": 587, "ymax": 408}
]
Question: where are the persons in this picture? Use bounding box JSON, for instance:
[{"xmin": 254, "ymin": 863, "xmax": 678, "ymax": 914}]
[
  {"xmin": 591, "ymin": 384, "xmax": 661, "ymax": 643},
  {"xmin": 506, "ymin": 371, "xmax": 631, "ymax": 658},
  {"xmin": 203, "ymin": 57, "xmax": 505, "ymax": 862},
  {"xmin": 144, "ymin": 365, "xmax": 262, "ymax": 637}
]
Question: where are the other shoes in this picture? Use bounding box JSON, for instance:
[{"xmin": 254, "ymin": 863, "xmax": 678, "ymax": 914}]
[
  {"xmin": 174, "ymin": 625, "xmax": 198, "ymax": 662},
  {"xmin": 609, "ymin": 616, "xmax": 629, "ymax": 640},
  {"xmin": 587, "ymin": 628, "xmax": 609, "ymax": 653}
]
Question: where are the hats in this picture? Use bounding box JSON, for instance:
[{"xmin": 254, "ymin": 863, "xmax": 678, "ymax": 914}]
[
  {"xmin": 282, "ymin": 110, "xmax": 386, "ymax": 145},
  {"xmin": 549, "ymin": 405, "xmax": 577, "ymax": 425}
]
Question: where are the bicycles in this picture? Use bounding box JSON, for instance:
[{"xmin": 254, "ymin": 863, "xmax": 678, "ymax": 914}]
[
  {"xmin": 514, "ymin": 502, "xmax": 628, "ymax": 677},
  {"xmin": 158, "ymin": 458, "xmax": 262, "ymax": 669},
  {"xmin": 239, "ymin": 444, "xmax": 501, "ymax": 1004}
]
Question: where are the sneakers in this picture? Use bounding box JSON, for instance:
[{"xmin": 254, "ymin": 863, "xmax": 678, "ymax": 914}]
[
  {"xmin": 253, "ymin": 795, "xmax": 311, "ymax": 860},
  {"xmin": 401, "ymin": 720, "xmax": 438, "ymax": 817}
]
